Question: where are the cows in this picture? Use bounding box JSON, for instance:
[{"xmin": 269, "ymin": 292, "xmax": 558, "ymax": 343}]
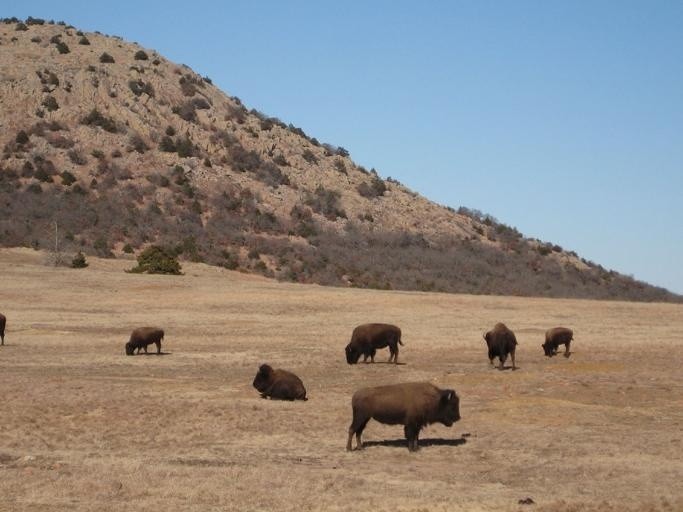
[
  {"xmin": 345, "ymin": 322, "xmax": 404, "ymax": 365},
  {"xmin": 125, "ymin": 326, "xmax": 164, "ymax": 355},
  {"xmin": 253, "ymin": 364, "xmax": 308, "ymax": 401},
  {"xmin": 483, "ymin": 323, "xmax": 518, "ymax": 370},
  {"xmin": 0, "ymin": 313, "xmax": 6, "ymax": 346},
  {"xmin": 346, "ymin": 381, "xmax": 460, "ymax": 453},
  {"xmin": 541, "ymin": 327, "xmax": 574, "ymax": 357}
]
